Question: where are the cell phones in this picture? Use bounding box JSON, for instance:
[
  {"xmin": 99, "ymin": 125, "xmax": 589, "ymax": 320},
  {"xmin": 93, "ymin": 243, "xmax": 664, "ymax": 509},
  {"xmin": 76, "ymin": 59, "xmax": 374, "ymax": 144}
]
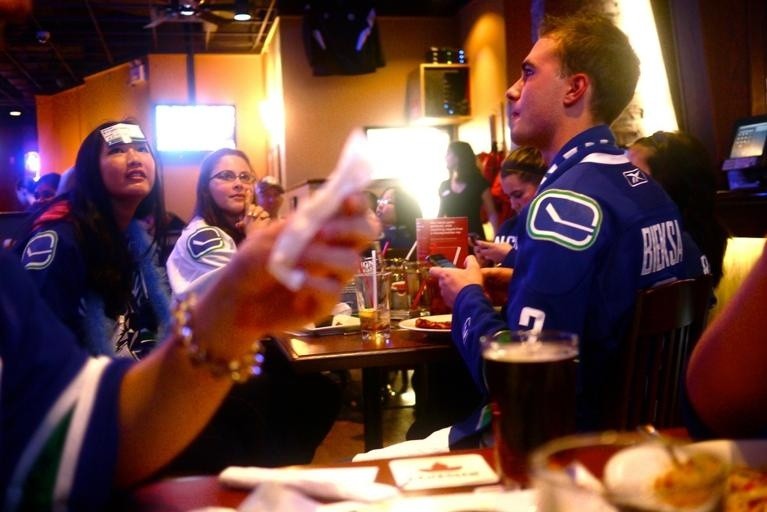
[
  {"xmin": 425, "ymin": 254, "xmax": 457, "ymax": 268},
  {"xmin": 467, "ymin": 231, "xmax": 481, "ymax": 245}
]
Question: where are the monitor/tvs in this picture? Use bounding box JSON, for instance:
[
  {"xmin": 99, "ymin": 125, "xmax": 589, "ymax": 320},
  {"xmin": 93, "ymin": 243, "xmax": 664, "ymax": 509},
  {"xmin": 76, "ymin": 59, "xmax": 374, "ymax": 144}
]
[
  {"xmin": 726, "ymin": 115, "xmax": 767, "ymax": 159},
  {"xmin": 153, "ymin": 103, "xmax": 237, "ymax": 156}
]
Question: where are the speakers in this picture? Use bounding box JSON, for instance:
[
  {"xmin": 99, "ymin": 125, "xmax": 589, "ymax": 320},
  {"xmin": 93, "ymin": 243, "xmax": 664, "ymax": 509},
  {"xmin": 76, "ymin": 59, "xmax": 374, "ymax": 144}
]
[
  {"xmin": 409, "ymin": 63, "xmax": 473, "ymax": 126},
  {"xmin": 302, "ymin": 0, "xmax": 386, "ymax": 77}
]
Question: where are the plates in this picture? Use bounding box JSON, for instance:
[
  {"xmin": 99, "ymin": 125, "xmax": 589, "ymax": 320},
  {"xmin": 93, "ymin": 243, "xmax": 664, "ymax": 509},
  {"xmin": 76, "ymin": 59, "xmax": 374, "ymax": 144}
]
[
  {"xmin": 302, "ymin": 314, "xmax": 360, "ymax": 335},
  {"xmin": 525, "ymin": 430, "xmax": 767, "ymax": 512},
  {"xmin": 399, "ymin": 314, "xmax": 453, "ymax": 334}
]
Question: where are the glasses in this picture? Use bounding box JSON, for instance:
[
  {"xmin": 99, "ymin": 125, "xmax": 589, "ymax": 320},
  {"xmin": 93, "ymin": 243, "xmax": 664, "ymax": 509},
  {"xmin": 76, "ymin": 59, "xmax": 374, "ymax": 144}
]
[
  {"xmin": 376, "ymin": 198, "xmax": 395, "ymax": 206},
  {"xmin": 208, "ymin": 170, "xmax": 257, "ymax": 184}
]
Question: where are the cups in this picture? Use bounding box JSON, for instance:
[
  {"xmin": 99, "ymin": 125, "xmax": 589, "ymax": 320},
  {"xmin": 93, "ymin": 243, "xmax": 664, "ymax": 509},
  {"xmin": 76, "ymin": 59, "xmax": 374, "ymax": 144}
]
[
  {"xmin": 403, "ymin": 260, "xmax": 432, "ymax": 316},
  {"xmin": 360, "ymin": 256, "xmax": 388, "ymax": 300},
  {"xmin": 479, "ymin": 332, "xmax": 581, "ymax": 485},
  {"xmin": 385, "ymin": 258, "xmax": 409, "ymax": 308},
  {"xmin": 355, "ymin": 272, "xmax": 391, "ymax": 337}
]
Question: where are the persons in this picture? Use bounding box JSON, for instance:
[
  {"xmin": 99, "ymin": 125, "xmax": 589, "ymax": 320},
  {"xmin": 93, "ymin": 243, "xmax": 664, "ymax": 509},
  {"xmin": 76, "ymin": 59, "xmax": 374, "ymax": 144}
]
[
  {"xmin": 347, "ymin": 5, "xmax": 713, "ymax": 466},
  {"xmin": 0, "ymin": 122, "xmax": 767, "ymax": 512}
]
